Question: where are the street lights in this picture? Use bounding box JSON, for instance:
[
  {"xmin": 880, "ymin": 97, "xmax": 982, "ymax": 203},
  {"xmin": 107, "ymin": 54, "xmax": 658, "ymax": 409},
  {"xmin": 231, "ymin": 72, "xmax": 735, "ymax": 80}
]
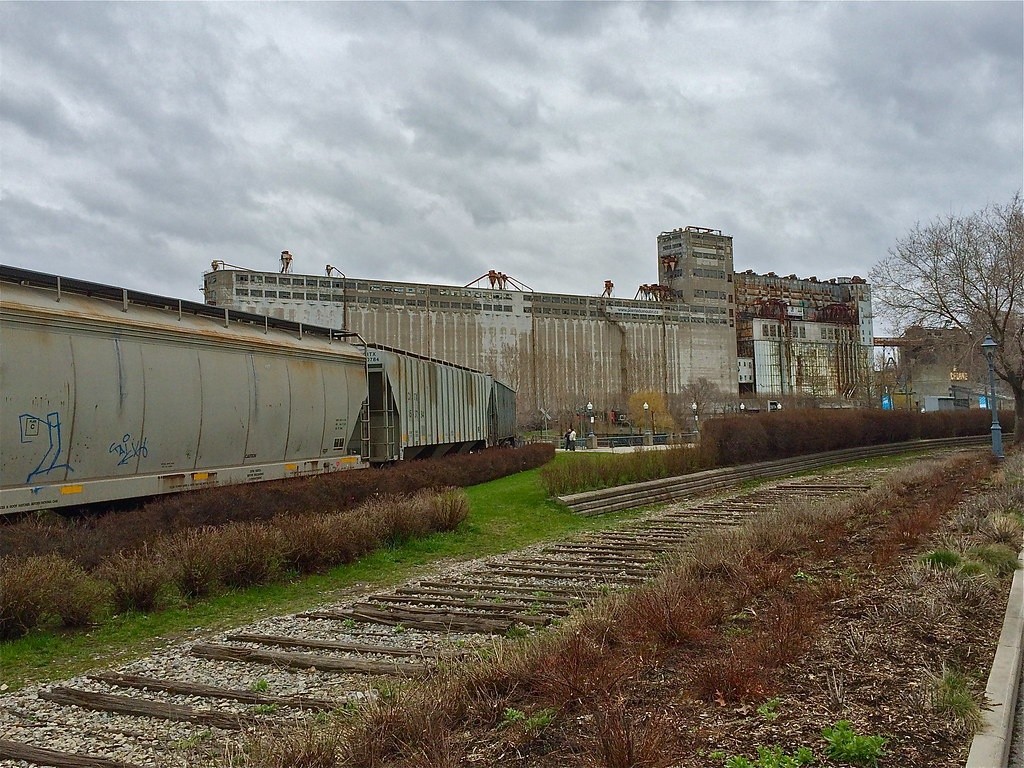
[
  {"xmin": 979, "ymin": 333, "xmax": 1004, "ymax": 459},
  {"xmin": 642, "ymin": 402, "xmax": 651, "ymax": 435},
  {"xmin": 587, "ymin": 401, "xmax": 595, "ymax": 437},
  {"xmin": 691, "ymin": 402, "xmax": 699, "ymax": 434}
]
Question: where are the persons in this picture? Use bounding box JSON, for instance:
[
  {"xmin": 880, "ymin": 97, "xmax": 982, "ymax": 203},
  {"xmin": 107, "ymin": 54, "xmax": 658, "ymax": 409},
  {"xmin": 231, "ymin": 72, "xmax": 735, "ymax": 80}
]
[
  {"xmin": 569, "ymin": 429, "xmax": 576, "ymax": 451},
  {"xmin": 564, "ymin": 429, "xmax": 572, "ymax": 451}
]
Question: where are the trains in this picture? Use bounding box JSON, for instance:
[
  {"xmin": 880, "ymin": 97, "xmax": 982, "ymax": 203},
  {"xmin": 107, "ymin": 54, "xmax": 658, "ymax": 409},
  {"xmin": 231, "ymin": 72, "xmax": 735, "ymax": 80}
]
[{"xmin": 0, "ymin": 264, "xmax": 520, "ymax": 530}]
